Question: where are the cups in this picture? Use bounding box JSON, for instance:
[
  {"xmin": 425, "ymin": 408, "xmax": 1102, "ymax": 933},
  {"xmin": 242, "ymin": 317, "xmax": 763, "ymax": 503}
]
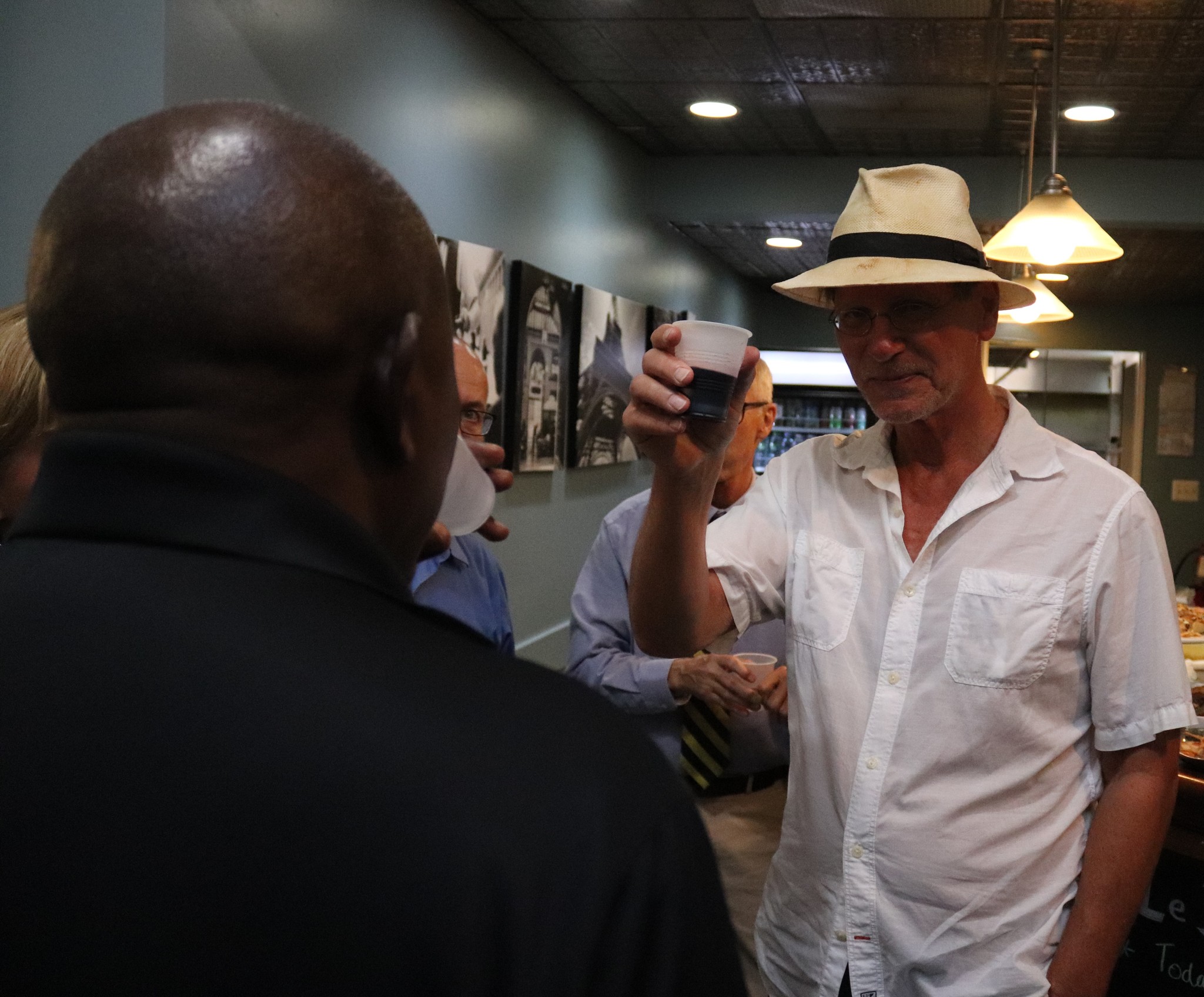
[
  {"xmin": 673, "ymin": 320, "xmax": 753, "ymax": 422},
  {"xmin": 733, "ymin": 652, "xmax": 777, "ymax": 714},
  {"xmin": 435, "ymin": 433, "xmax": 497, "ymax": 537}
]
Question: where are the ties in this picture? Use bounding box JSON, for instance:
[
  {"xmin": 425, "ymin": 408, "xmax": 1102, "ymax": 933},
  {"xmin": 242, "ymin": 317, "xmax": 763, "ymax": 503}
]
[{"xmin": 679, "ymin": 506, "xmax": 734, "ymax": 794}]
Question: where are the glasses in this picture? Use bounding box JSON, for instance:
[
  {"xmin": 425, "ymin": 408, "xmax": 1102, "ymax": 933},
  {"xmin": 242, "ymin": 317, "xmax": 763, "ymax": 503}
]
[
  {"xmin": 826, "ymin": 290, "xmax": 964, "ymax": 337},
  {"xmin": 736, "ymin": 400, "xmax": 769, "ymax": 424},
  {"xmin": 459, "ymin": 409, "xmax": 497, "ymax": 437}
]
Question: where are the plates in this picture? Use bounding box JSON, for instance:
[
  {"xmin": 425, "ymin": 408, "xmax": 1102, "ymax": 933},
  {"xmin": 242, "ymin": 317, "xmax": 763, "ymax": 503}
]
[{"xmin": 1176, "ymin": 605, "xmax": 1204, "ymax": 669}]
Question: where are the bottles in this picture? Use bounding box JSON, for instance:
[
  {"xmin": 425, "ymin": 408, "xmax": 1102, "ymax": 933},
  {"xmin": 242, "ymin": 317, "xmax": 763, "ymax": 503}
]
[
  {"xmin": 773, "ymin": 398, "xmax": 867, "ymax": 432},
  {"xmin": 754, "ymin": 433, "xmax": 816, "ymax": 467}
]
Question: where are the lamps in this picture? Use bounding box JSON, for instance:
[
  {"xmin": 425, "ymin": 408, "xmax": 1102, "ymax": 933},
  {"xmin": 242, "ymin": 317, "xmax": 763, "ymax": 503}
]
[
  {"xmin": 983, "ymin": 0, "xmax": 1124, "ymax": 264},
  {"xmin": 996, "ymin": 48, "xmax": 1075, "ymax": 324}
]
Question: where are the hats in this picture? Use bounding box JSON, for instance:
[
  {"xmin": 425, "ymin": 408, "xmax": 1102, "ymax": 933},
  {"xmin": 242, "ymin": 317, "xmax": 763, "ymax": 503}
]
[{"xmin": 771, "ymin": 162, "xmax": 1036, "ymax": 312}]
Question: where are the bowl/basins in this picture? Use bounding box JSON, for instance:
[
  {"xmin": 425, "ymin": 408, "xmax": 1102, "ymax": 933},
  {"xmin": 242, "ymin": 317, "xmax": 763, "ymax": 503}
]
[{"xmin": 1177, "ymin": 685, "xmax": 1204, "ymax": 765}]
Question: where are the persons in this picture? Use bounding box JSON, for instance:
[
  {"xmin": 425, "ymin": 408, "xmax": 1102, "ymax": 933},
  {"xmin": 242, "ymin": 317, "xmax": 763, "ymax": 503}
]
[
  {"xmin": 0, "ymin": 95, "xmax": 754, "ymax": 997},
  {"xmin": 571, "ymin": 354, "xmax": 793, "ymax": 997},
  {"xmin": 623, "ymin": 161, "xmax": 1200, "ymax": 997}
]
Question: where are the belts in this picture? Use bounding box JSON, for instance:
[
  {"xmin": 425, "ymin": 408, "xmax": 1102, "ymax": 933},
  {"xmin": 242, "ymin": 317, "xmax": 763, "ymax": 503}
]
[{"xmin": 697, "ymin": 763, "xmax": 789, "ymax": 798}]
